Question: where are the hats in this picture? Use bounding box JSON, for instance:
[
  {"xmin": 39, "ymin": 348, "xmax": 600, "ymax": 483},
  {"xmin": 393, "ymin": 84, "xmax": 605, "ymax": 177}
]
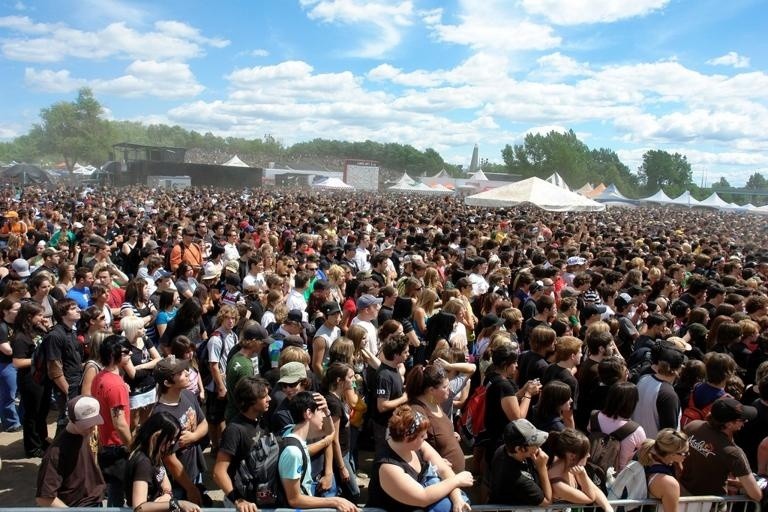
[
  {"xmin": 152, "ymin": 267, "xmax": 174, "ymax": 282},
  {"xmin": 66, "ymin": 394, "xmax": 105, "ymax": 429},
  {"xmin": 322, "ymin": 301, "xmax": 341, "ymax": 316},
  {"xmin": 152, "ymin": 357, "xmax": 192, "ymax": 381},
  {"xmin": 12, "ymin": 258, "xmax": 31, "ymax": 277},
  {"xmin": 482, "ymin": 312, "xmax": 508, "ymax": 332},
  {"xmin": 356, "ymin": 294, "xmax": 383, "ymax": 310},
  {"xmin": 276, "ymin": 361, "xmax": 308, "ymax": 384},
  {"xmin": 614, "ymin": 293, "xmax": 638, "ymax": 308},
  {"xmin": 241, "ymin": 323, "xmax": 275, "ymax": 345}
]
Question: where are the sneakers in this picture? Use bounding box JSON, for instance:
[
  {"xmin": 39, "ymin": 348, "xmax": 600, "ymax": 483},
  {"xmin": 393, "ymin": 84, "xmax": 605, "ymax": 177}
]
[{"xmin": 24, "ymin": 448, "xmax": 44, "ymax": 457}]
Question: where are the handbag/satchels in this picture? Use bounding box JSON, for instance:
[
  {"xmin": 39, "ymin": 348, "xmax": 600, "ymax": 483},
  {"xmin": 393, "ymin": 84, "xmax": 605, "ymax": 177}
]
[{"xmin": 420, "ymin": 465, "xmax": 471, "ymax": 512}]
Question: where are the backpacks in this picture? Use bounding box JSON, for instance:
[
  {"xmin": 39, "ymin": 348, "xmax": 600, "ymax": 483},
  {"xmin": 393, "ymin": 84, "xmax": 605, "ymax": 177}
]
[
  {"xmin": 606, "ymin": 450, "xmax": 675, "ymax": 512},
  {"xmin": 578, "ymin": 410, "xmax": 640, "ymax": 498},
  {"xmin": 234, "ymin": 426, "xmax": 308, "ymax": 507}
]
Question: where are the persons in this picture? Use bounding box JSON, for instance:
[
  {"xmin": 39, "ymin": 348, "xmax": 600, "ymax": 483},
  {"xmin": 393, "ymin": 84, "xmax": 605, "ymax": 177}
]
[{"xmin": 0, "ymin": 146, "xmax": 768, "ymax": 511}]
